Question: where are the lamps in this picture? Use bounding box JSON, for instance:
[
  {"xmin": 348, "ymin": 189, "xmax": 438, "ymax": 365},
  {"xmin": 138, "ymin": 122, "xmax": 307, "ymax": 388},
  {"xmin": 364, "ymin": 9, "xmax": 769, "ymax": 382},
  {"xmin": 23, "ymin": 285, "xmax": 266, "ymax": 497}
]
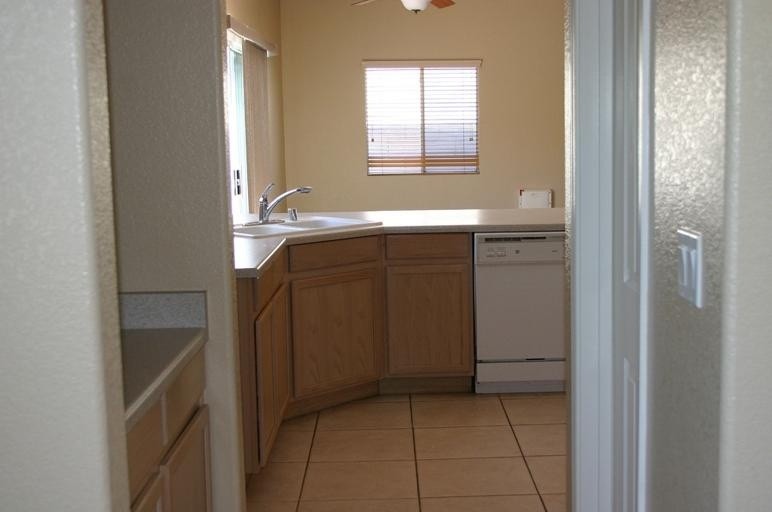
[{"xmin": 349, "ymin": 0, "xmax": 455, "ymax": 14}]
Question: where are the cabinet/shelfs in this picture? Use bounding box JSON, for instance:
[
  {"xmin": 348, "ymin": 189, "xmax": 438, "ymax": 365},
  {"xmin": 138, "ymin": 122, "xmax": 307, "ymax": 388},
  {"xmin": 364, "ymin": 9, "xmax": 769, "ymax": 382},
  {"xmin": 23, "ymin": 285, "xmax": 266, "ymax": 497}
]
[
  {"xmin": 237, "ymin": 246, "xmax": 293, "ymax": 475},
  {"xmin": 128, "ymin": 346, "xmax": 213, "ymax": 511},
  {"xmin": 382, "ymin": 233, "xmax": 474, "ymax": 395},
  {"xmin": 290, "ymin": 234, "xmax": 382, "ymax": 418}
]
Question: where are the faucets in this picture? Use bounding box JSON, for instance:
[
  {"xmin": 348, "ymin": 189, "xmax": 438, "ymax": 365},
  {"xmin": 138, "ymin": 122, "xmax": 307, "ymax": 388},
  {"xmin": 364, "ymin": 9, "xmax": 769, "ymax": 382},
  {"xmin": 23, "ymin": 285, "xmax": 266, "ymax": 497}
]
[{"xmin": 255, "ymin": 182, "xmax": 313, "ymax": 224}]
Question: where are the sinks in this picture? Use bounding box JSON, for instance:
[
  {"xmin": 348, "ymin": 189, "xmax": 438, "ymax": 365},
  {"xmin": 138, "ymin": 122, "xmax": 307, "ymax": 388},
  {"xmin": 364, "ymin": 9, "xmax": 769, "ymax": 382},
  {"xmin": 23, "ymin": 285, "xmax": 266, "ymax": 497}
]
[
  {"xmin": 233, "ymin": 224, "xmax": 305, "ymax": 237},
  {"xmin": 287, "ymin": 215, "xmax": 383, "ymax": 229}
]
